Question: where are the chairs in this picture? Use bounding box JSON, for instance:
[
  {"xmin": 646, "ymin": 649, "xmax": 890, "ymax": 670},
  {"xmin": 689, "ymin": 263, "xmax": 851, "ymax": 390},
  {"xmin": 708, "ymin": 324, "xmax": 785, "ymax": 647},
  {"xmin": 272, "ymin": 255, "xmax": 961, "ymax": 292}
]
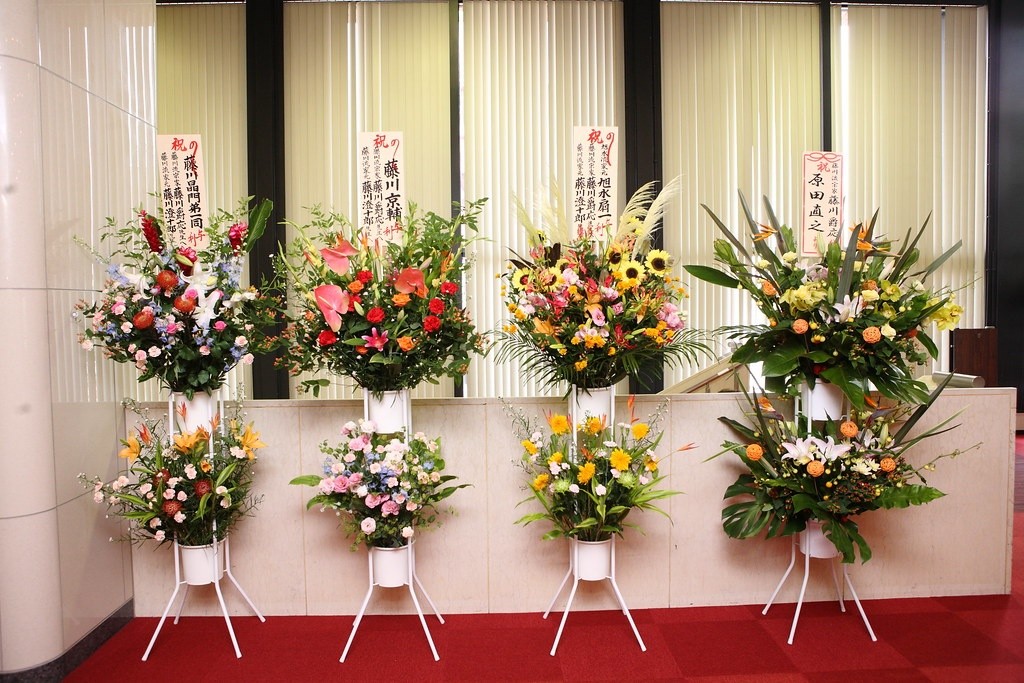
[{"xmin": 657, "ymin": 353, "xmax": 750, "ymax": 394}]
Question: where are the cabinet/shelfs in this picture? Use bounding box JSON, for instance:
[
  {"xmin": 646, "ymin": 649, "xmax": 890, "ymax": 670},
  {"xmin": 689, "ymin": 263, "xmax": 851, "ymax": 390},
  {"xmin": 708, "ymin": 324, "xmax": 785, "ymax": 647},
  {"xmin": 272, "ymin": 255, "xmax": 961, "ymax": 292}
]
[{"xmin": 948, "ymin": 327, "xmax": 999, "ymax": 388}]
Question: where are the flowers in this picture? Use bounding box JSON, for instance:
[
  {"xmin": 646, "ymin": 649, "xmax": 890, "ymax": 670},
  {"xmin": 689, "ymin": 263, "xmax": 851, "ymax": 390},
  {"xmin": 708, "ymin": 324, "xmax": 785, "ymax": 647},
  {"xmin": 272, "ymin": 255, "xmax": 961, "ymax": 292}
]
[
  {"xmin": 682, "ymin": 186, "xmax": 984, "ymax": 414},
  {"xmin": 700, "ymin": 368, "xmax": 983, "ymax": 566},
  {"xmin": 69, "ymin": 190, "xmax": 287, "ymax": 402},
  {"xmin": 483, "ymin": 176, "xmax": 728, "ymax": 409},
  {"xmin": 497, "ymin": 392, "xmax": 685, "ymax": 543},
  {"xmin": 74, "ymin": 380, "xmax": 270, "ymax": 552},
  {"xmin": 268, "ymin": 197, "xmax": 491, "ymax": 409},
  {"xmin": 288, "ymin": 416, "xmax": 475, "ymax": 553}
]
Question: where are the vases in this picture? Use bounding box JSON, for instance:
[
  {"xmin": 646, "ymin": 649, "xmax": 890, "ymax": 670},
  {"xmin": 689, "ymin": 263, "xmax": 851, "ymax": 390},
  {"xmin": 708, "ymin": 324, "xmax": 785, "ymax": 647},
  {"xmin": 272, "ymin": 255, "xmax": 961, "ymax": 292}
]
[
  {"xmin": 566, "ymin": 381, "xmax": 611, "ymax": 429},
  {"xmin": 800, "ymin": 378, "xmax": 843, "ymax": 421},
  {"xmin": 172, "ymin": 387, "xmax": 221, "ymax": 433},
  {"xmin": 799, "ymin": 518, "xmax": 842, "ymax": 559},
  {"xmin": 567, "ymin": 536, "xmax": 612, "ymax": 581},
  {"xmin": 368, "ymin": 390, "xmax": 407, "ymax": 434},
  {"xmin": 372, "ymin": 535, "xmax": 416, "ymax": 588},
  {"xmin": 178, "ymin": 536, "xmax": 227, "ymax": 586}
]
